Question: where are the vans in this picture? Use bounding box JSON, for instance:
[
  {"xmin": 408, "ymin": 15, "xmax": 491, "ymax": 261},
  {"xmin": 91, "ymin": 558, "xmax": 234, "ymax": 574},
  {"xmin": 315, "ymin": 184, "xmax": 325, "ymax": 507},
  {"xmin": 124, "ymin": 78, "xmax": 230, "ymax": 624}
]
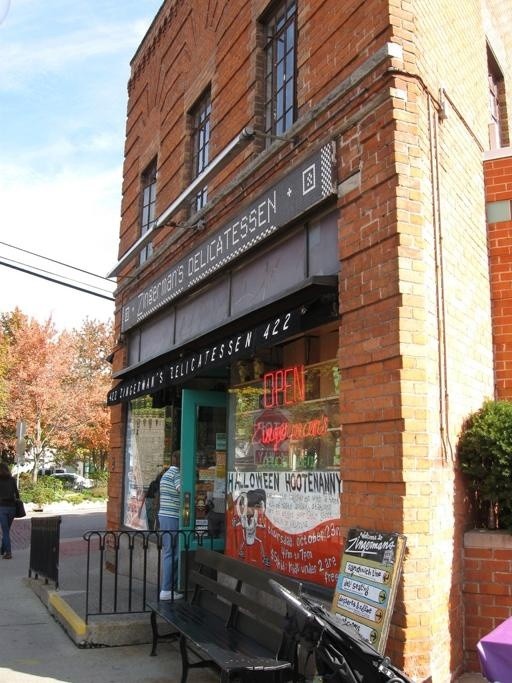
[{"xmin": 26, "ymin": 469, "xmax": 67, "ymax": 477}]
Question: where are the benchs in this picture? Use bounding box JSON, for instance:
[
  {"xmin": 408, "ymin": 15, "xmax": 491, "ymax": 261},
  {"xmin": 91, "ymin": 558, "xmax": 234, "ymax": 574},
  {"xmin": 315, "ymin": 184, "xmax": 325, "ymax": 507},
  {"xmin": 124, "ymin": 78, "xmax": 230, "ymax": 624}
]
[{"xmin": 148, "ymin": 547, "xmax": 301, "ymax": 683}]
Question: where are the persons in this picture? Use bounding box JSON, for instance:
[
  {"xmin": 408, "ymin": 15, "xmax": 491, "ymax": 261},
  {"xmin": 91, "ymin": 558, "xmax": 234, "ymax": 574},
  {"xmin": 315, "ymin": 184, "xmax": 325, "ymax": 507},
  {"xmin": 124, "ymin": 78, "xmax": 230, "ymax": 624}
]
[
  {"xmin": 0, "ymin": 463, "xmax": 20, "ymax": 559},
  {"xmin": 138, "ymin": 475, "xmax": 160, "ymax": 534},
  {"xmin": 159, "ymin": 450, "xmax": 184, "ymax": 601}
]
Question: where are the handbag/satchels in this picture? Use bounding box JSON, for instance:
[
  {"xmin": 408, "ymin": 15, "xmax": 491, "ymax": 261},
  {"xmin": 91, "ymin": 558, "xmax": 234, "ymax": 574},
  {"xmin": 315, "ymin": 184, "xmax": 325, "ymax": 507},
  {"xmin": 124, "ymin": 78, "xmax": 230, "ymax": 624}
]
[
  {"xmin": 145, "ymin": 468, "xmax": 169, "ymax": 532},
  {"xmin": 15, "ymin": 499, "xmax": 25, "ymax": 518}
]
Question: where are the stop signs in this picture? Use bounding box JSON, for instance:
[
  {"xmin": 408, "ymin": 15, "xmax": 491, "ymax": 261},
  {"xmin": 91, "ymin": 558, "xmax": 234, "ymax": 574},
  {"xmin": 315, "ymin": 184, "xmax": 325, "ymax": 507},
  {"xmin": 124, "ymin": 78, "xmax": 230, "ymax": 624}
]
[{"xmin": 252, "ymin": 410, "xmax": 293, "ymax": 450}]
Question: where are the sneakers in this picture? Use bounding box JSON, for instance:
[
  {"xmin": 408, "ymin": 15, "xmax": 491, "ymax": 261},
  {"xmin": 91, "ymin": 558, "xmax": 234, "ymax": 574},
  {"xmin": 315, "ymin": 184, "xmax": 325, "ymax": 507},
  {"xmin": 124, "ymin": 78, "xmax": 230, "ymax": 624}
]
[
  {"xmin": 159, "ymin": 590, "xmax": 184, "ymax": 601},
  {"xmin": 3, "ymin": 554, "xmax": 12, "ymax": 560}
]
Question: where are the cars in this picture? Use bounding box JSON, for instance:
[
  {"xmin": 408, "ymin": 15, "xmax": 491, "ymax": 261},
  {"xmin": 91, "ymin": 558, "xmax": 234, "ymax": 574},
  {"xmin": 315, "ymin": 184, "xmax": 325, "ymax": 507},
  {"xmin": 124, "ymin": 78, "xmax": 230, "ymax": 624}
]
[{"xmin": 47, "ymin": 474, "xmax": 94, "ymax": 491}]
